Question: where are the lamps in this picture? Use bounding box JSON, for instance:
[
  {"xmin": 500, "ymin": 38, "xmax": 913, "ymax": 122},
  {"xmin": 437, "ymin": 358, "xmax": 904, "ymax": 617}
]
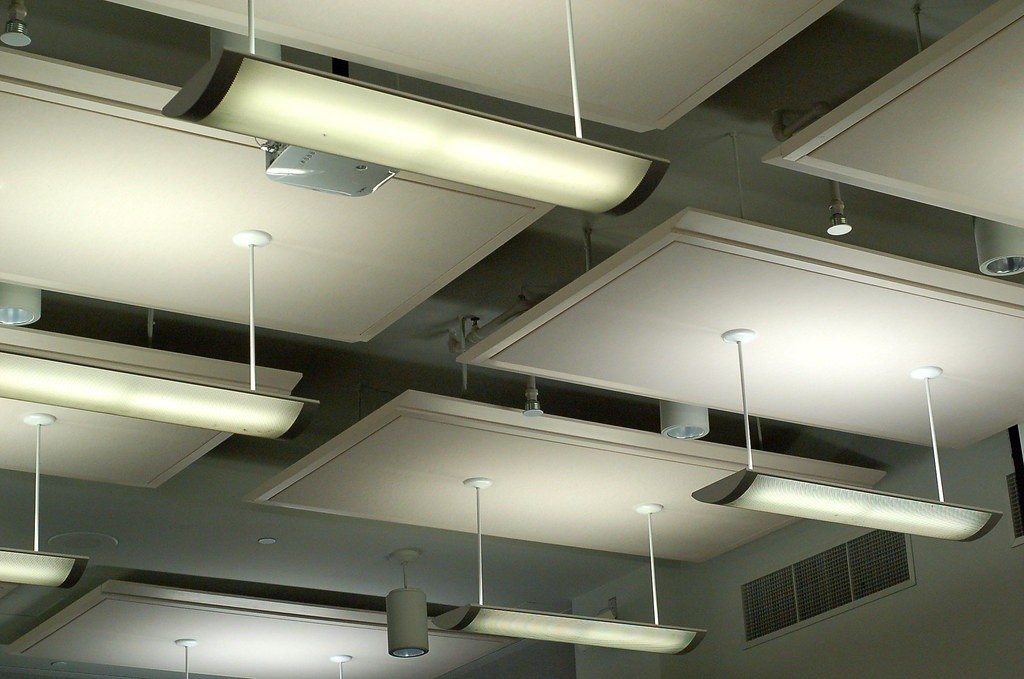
[
  {"xmin": 523, "ymin": 373, "xmax": 545, "ymax": 416},
  {"xmin": 384, "ymin": 548, "xmax": 430, "ymax": 659},
  {"xmin": 431, "ymin": 475, "xmax": 709, "ymax": 655},
  {"xmin": 825, "ymin": 177, "xmax": 854, "ymax": 237},
  {"xmin": 0, "ymin": 231, "xmax": 320, "ymax": 441},
  {"xmin": 973, "ymin": 217, "xmax": 1024, "ymax": 276},
  {"xmin": 0, "ymin": 281, "xmax": 41, "ymax": 325},
  {"xmin": 161, "ymin": 0, "xmax": 672, "ymax": 216},
  {"xmin": 691, "ymin": 328, "xmax": 1004, "ymax": 543},
  {"xmin": 658, "ymin": 403, "xmax": 710, "ymax": 441},
  {"xmin": 0, "ymin": 412, "xmax": 90, "ymax": 588},
  {"xmin": 0, "ymin": 0, "xmax": 31, "ymax": 47}
]
